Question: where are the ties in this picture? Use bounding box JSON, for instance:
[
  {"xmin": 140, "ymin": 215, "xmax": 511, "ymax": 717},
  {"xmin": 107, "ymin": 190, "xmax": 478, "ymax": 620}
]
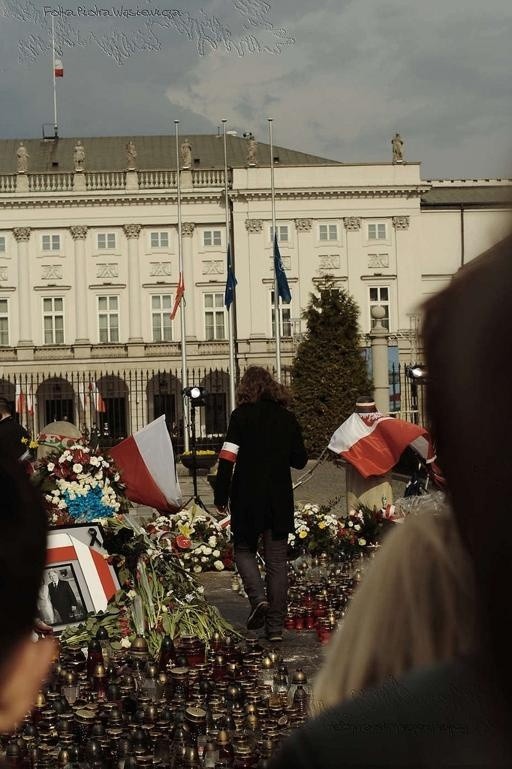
[{"xmin": 54, "ymin": 583, "xmax": 57, "ymax": 591}]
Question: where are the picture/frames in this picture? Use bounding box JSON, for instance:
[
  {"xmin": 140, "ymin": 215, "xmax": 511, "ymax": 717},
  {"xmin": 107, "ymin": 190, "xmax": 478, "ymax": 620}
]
[{"xmin": 37, "ymin": 522, "xmax": 122, "ymax": 640}]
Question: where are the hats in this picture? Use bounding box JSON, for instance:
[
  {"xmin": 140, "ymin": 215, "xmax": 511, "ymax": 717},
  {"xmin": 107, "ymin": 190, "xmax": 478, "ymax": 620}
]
[{"xmin": 355, "ymin": 396, "xmax": 378, "ymax": 413}]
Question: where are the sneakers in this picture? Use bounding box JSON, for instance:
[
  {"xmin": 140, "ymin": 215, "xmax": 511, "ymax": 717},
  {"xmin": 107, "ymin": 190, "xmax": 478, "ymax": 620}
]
[
  {"xmin": 268, "ymin": 633, "xmax": 283, "ymax": 641},
  {"xmin": 247, "ymin": 599, "xmax": 271, "ymax": 630}
]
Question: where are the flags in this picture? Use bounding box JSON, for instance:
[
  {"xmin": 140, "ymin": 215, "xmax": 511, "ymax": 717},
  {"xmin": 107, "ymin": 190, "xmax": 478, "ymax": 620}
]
[
  {"xmin": 274, "ymin": 232, "xmax": 291, "ymax": 304},
  {"xmin": 170, "ymin": 273, "xmax": 184, "ymax": 320},
  {"xmin": 225, "ymin": 243, "xmax": 237, "ymax": 311}
]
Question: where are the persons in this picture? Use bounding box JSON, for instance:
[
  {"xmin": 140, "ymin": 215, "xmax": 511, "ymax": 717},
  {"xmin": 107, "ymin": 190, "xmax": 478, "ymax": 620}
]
[
  {"xmin": 37, "ymin": 576, "xmax": 54, "ymax": 626},
  {"xmin": 308, "ymin": 510, "xmax": 476, "ymax": 718},
  {"xmin": 391, "ymin": 133, "xmax": 403, "ymax": 161},
  {"xmin": 48, "ymin": 569, "xmax": 78, "ymax": 623},
  {"xmin": 265, "ymin": 255, "xmax": 512, "ymax": 769},
  {"xmin": 0, "ymin": 398, "xmax": 31, "ymax": 460},
  {"xmin": 126, "ymin": 141, "xmax": 136, "ymax": 167},
  {"xmin": 247, "ymin": 135, "xmax": 256, "ymax": 162},
  {"xmin": 0, "ymin": 471, "xmax": 59, "ymax": 738},
  {"xmin": 82, "ymin": 421, "xmax": 112, "ymax": 439},
  {"xmin": 72, "ymin": 140, "xmax": 85, "ymax": 168},
  {"xmin": 214, "ymin": 366, "xmax": 308, "ymax": 642},
  {"xmin": 180, "ymin": 139, "xmax": 192, "ymax": 165},
  {"xmin": 16, "ymin": 141, "xmax": 29, "ymax": 171}
]
